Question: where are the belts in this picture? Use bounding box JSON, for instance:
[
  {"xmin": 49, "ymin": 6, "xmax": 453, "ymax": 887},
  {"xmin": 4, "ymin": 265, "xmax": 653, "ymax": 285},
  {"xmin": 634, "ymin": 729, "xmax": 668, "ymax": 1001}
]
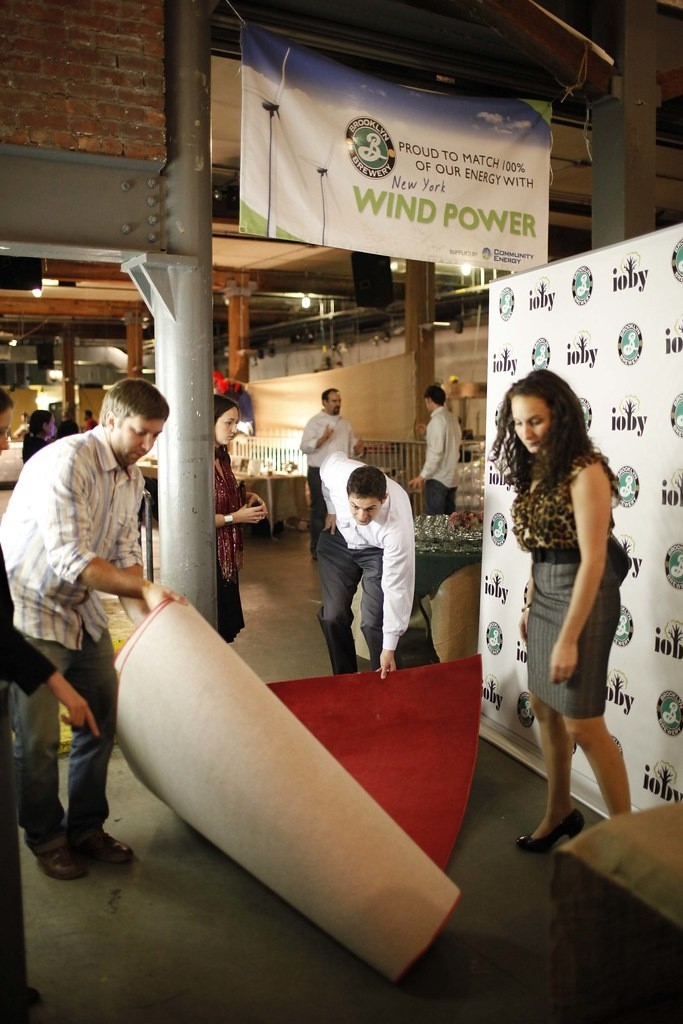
[{"xmin": 531, "ymin": 548, "xmax": 581, "ymax": 564}]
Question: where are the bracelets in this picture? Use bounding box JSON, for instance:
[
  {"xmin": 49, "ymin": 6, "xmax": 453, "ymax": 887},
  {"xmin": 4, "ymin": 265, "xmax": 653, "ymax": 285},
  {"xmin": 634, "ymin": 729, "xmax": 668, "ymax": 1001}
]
[
  {"xmin": 522, "ymin": 603, "xmax": 530, "ymax": 612},
  {"xmin": 223, "ymin": 513, "xmax": 233, "ymax": 526}
]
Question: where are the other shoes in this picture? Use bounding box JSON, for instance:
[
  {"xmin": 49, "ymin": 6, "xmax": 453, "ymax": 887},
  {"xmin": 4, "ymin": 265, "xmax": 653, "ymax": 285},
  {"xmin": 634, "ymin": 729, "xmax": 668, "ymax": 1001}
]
[{"xmin": 311, "ymin": 548, "xmax": 318, "ymax": 561}]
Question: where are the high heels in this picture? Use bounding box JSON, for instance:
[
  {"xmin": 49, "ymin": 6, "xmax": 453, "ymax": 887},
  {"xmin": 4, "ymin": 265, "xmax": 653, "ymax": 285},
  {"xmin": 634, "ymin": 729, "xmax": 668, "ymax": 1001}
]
[{"xmin": 515, "ymin": 808, "xmax": 586, "ymax": 854}]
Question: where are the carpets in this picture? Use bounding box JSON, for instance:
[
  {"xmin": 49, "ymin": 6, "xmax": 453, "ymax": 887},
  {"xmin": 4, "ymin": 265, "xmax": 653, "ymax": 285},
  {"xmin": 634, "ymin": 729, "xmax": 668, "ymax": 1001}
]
[{"xmin": 106, "ymin": 593, "xmax": 481, "ymax": 995}]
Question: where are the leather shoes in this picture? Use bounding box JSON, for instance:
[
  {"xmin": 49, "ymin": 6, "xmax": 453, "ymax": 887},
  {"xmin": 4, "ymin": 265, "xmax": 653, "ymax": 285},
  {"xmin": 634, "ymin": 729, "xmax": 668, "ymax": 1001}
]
[
  {"xmin": 76, "ymin": 831, "xmax": 134, "ymax": 863},
  {"xmin": 39, "ymin": 845, "xmax": 83, "ymax": 881}
]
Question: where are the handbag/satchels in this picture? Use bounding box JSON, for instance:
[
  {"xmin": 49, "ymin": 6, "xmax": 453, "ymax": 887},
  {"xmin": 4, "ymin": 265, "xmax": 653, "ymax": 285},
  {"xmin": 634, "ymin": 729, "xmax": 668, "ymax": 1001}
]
[{"xmin": 607, "ymin": 534, "xmax": 632, "ymax": 585}]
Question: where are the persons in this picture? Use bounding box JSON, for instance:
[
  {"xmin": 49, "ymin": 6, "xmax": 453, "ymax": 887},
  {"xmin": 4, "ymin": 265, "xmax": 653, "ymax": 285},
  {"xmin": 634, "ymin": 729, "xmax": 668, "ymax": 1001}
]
[
  {"xmin": 487, "ymin": 369, "xmax": 632, "ymax": 852},
  {"xmin": 408, "ymin": 385, "xmax": 463, "ymax": 515},
  {"xmin": 0, "ymin": 378, "xmax": 189, "ymax": 1024},
  {"xmin": 316, "ymin": 451, "xmax": 415, "ymax": 679},
  {"xmin": 214, "ymin": 393, "xmax": 268, "ymax": 644},
  {"xmin": 300, "ymin": 389, "xmax": 364, "ymax": 561}
]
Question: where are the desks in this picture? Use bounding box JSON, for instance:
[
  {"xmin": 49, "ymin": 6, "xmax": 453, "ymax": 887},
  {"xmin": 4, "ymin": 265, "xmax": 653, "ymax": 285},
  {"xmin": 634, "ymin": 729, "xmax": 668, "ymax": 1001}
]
[
  {"xmin": 137, "ymin": 460, "xmax": 307, "ymax": 542},
  {"xmin": 414, "ymin": 539, "xmax": 482, "ymax": 647}
]
